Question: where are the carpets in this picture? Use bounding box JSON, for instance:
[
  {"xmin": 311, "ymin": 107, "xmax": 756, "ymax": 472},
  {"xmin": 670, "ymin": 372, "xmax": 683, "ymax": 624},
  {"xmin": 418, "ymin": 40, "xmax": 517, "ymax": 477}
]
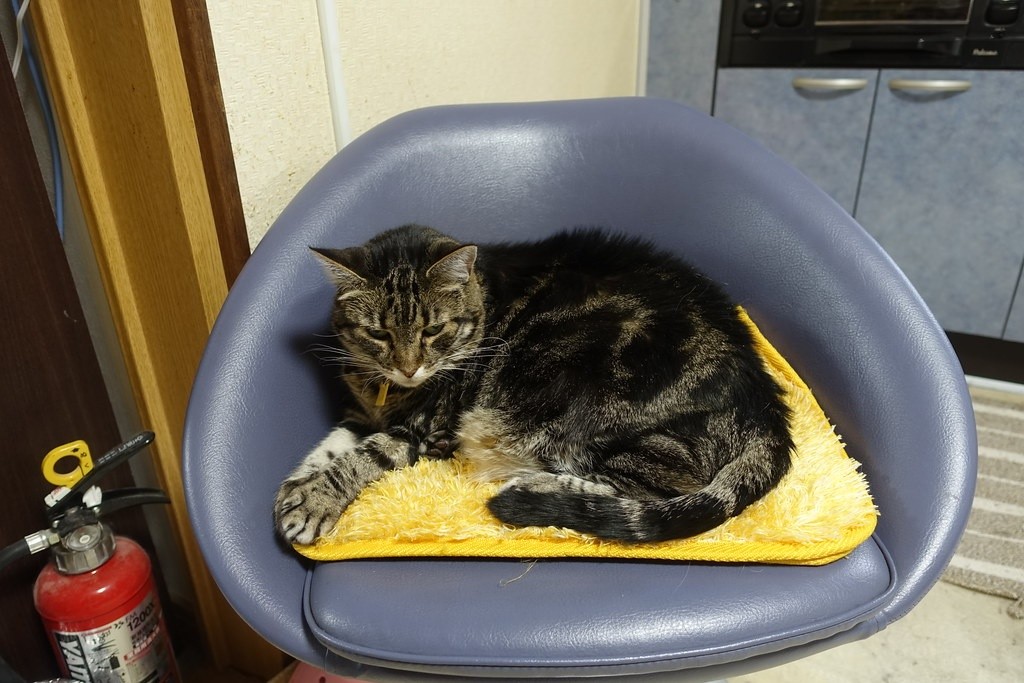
[{"xmin": 934, "ymin": 382, "xmax": 1023, "ymax": 620}]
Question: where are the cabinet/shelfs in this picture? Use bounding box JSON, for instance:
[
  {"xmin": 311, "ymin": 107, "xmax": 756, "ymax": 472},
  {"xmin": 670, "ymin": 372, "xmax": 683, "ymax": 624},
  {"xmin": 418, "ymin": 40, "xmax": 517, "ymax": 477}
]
[
  {"xmin": 709, "ymin": 68, "xmax": 1024, "ymax": 344},
  {"xmin": 1000, "ymin": 262, "xmax": 1023, "ymax": 344}
]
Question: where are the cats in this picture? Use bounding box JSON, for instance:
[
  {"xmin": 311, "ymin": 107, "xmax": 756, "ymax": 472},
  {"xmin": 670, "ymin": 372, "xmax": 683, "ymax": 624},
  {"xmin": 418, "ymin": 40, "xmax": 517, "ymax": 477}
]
[{"xmin": 273, "ymin": 222, "xmax": 799, "ymax": 546}]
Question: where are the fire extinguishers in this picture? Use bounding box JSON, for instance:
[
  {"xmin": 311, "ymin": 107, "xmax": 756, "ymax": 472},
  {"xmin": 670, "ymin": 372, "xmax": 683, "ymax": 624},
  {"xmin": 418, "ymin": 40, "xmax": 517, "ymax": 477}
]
[{"xmin": 0, "ymin": 430, "xmax": 182, "ymax": 683}]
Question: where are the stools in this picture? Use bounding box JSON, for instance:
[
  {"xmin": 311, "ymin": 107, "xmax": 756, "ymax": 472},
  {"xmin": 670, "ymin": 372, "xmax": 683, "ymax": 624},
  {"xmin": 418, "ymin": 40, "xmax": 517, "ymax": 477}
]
[{"xmin": 181, "ymin": 93, "xmax": 980, "ymax": 681}]
